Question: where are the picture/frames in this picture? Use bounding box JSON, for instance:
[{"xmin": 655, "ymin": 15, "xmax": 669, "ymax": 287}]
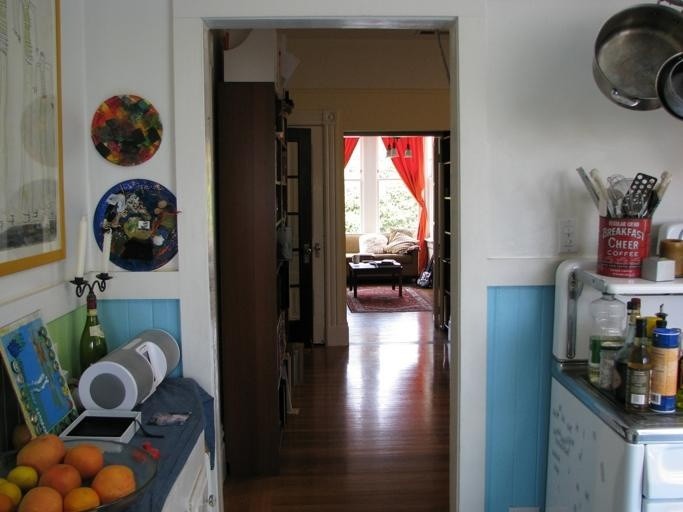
[{"xmin": 0, "ymin": 0, "xmax": 66, "ymax": 276}]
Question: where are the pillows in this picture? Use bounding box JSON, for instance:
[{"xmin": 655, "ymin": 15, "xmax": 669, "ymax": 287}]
[{"xmin": 359, "ymin": 227, "xmax": 420, "ymax": 254}]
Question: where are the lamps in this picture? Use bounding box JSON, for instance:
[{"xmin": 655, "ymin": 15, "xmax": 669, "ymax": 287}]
[{"xmin": 385, "ymin": 137, "xmax": 413, "ymax": 159}]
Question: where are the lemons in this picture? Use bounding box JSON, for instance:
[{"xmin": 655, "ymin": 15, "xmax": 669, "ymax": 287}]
[
  {"xmin": 0, "ymin": 479, "xmax": 6, "ymax": 488},
  {"xmin": 8, "ymin": 466, "xmax": 37, "ymax": 488},
  {"xmin": 0, "ymin": 484, "xmax": 21, "ymax": 504}
]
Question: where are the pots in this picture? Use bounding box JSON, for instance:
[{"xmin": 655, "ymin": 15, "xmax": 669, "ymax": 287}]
[{"xmin": 592, "ymin": 0, "xmax": 683, "ymax": 119}]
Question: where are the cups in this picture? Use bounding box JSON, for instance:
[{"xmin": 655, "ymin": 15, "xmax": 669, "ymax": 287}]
[
  {"xmin": 351, "ymin": 254, "xmax": 360, "ymax": 265},
  {"xmin": 660, "ymin": 239, "xmax": 683, "ymax": 278}
]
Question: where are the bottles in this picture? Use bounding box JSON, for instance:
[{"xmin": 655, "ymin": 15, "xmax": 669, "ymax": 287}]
[
  {"xmin": 80, "ymin": 294, "xmax": 107, "ymax": 373},
  {"xmin": 588, "ymin": 292, "xmax": 683, "ymax": 413}
]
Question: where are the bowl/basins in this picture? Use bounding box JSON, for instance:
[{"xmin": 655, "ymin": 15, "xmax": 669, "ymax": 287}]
[{"xmin": 0, "ymin": 438, "xmax": 159, "ymax": 512}]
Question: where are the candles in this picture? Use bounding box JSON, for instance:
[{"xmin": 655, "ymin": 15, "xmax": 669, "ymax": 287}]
[
  {"xmin": 100, "ymin": 229, "xmax": 112, "ymax": 273},
  {"xmin": 76, "ymin": 215, "xmax": 88, "ymax": 278}
]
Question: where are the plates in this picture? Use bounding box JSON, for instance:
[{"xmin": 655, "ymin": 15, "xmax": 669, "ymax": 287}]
[
  {"xmin": 90, "ymin": 94, "xmax": 164, "ymax": 166},
  {"xmin": 93, "ymin": 178, "xmax": 178, "ymax": 272}
]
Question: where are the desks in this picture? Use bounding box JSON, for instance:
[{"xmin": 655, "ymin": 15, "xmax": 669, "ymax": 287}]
[{"xmin": 348, "ymin": 262, "xmax": 403, "ymax": 298}]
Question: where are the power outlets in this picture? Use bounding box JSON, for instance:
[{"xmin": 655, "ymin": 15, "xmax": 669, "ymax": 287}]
[{"xmin": 559, "ymin": 218, "xmax": 580, "ymax": 254}]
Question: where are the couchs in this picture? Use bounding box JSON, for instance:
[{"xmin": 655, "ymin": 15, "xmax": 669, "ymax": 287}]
[{"xmin": 345, "ymin": 233, "xmax": 422, "ymax": 288}]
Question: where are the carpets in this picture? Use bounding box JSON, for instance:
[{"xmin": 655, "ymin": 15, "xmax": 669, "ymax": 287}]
[{"xmin": 346, "ymin": 285, "xmax": 434, "ymax": 313}]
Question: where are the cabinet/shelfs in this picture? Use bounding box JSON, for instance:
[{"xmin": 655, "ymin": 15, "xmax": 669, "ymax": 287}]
[
  {"xmin": 216, "ymin": 83, "xmax": 288, "ymax": 477},
  {"xmin": 438, "ymin": 134, "xmax": 451, "ymax": 328},
  {"xmin": 162, "ymin": 426, "xmax": 221, "ymax": 512}
]
